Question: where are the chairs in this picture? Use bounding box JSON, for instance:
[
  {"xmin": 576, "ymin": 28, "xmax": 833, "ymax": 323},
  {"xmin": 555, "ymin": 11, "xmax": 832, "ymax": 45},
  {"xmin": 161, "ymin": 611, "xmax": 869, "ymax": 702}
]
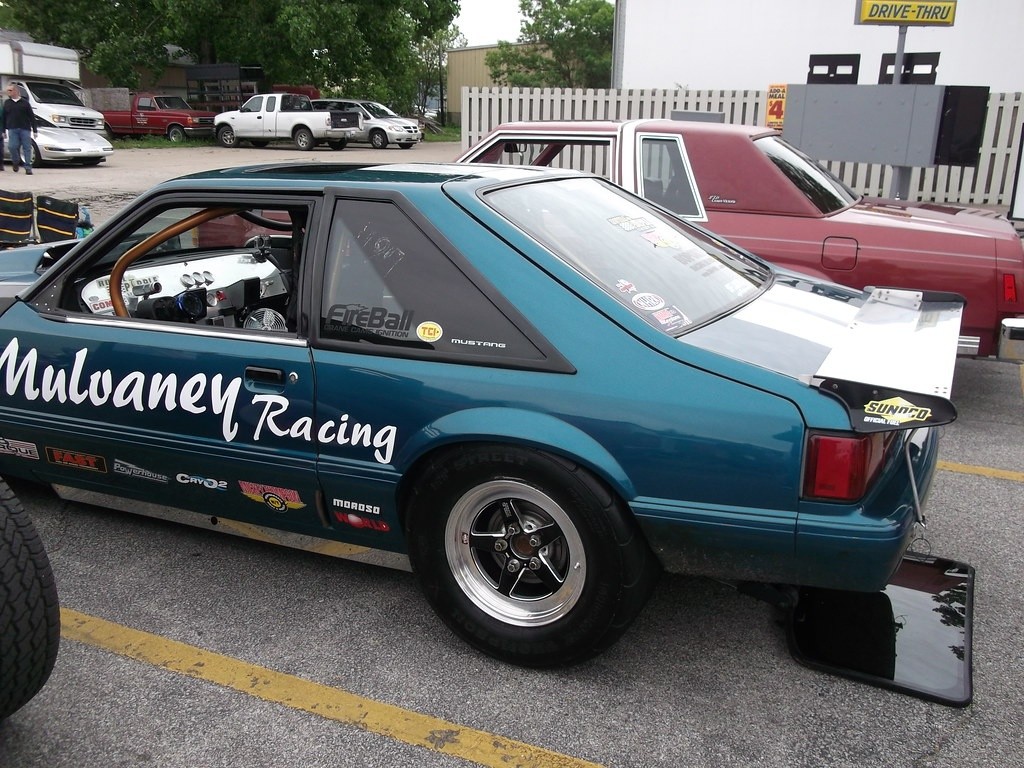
[{"xmin": 643, "ymin": 177, "xmax": 664, "ymax": 200}]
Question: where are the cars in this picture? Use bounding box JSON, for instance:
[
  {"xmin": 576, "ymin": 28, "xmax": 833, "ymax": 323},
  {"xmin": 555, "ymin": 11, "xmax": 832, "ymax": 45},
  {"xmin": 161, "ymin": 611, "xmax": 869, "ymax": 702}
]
[
  {"xmin": 0, "ymin": 158, "xmax": 967, "ymax": 672},
  {"xmin": 1, "ymin": 108, "xmax": 116, "ymax": 168},
  {"xmin": 190, "ymin": 116, "xmax": 1023, "ymax": 360}
]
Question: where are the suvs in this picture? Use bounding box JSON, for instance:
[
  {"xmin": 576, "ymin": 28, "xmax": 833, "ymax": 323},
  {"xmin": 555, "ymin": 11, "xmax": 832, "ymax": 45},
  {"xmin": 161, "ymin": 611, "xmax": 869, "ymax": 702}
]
[{"xmin": 301, "ymin": 98, "xmax": 424, "ymax": 150}]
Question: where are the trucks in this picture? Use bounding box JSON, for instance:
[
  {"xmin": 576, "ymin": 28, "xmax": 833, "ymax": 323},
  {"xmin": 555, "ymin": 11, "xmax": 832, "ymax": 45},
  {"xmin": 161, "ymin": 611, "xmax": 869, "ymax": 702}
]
[{"xmin": 0, "ymin": 39, "xmax": 107, "ymax": 137}]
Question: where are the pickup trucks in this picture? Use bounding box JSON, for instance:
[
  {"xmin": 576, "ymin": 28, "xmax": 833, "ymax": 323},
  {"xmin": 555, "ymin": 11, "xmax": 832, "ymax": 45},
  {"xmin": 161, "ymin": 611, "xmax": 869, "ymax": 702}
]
[
  {"xmin": 211, "ymin": 93, "xmax": 366, "ymax": 151},
  {"xmin": 97, "ymin": 92, "xmax": 220, "ymax": 145}
]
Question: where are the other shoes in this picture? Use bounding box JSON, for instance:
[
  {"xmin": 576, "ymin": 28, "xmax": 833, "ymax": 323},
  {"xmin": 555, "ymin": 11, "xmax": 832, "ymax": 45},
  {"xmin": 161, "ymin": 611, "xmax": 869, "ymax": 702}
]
[
  {"xmin": 0, "ymin": 163, "xmax": 4, "ymax": 171},
  {"xmin": 13, "ymin": 161, "xmax": 19, "ymax": 172},
  {"xmin": 26, "ymin": 169, "xmax": 32, "ymax": 175}
]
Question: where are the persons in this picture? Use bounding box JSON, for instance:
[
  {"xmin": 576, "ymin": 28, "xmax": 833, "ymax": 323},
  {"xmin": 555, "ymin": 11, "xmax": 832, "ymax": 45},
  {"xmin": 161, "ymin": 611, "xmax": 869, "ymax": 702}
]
[{"xmin": 0, "ymin": 84, "xmax": 38, "ymax": 175}]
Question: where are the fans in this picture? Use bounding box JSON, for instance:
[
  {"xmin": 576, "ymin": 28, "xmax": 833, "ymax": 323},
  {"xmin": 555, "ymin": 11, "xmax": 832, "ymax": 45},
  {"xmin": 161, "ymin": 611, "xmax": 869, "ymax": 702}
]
[{"xmin": 243, "ymin": 308, "xmax": 288, "ymax": 333}]
